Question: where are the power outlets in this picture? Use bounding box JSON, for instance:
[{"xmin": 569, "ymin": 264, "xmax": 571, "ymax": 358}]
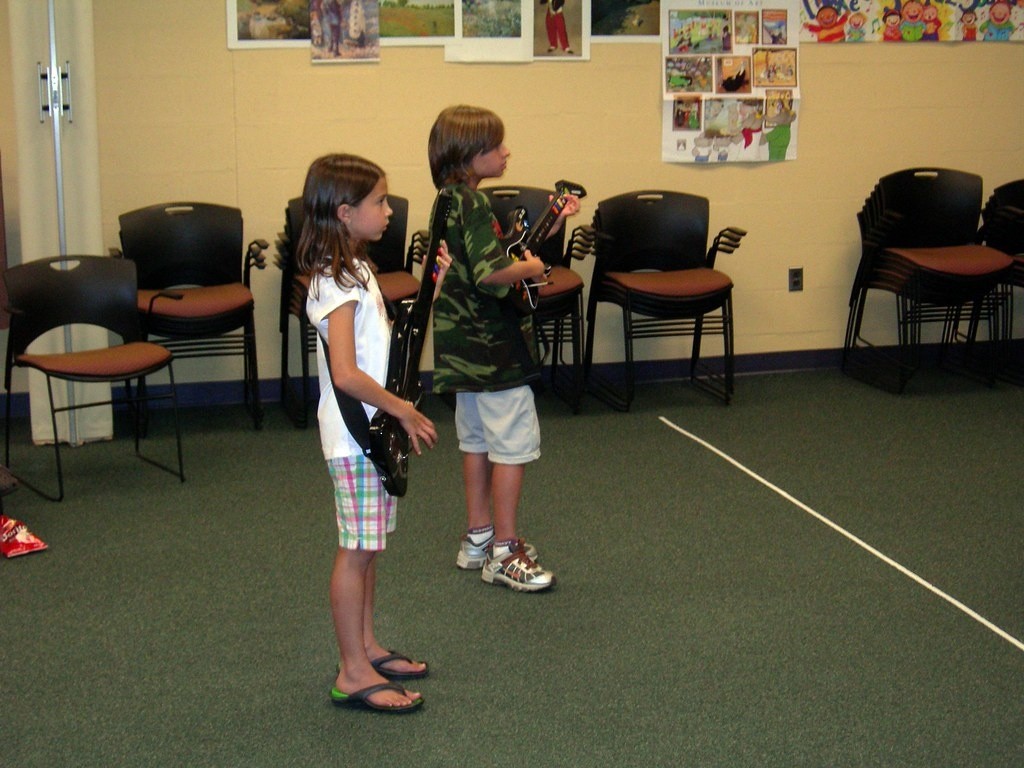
[{"xmin": 788, "ymin": 267, "xmax": 803, "ymax": 292}]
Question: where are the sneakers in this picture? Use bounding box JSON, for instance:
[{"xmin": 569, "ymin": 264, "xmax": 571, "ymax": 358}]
[
  {"xmin": 481, "ymin": 538, "xmax": 555, "ymax": 591},
  {"xmin": 456, "ymin": 531, "xmax": 539, "ymax": 569}
]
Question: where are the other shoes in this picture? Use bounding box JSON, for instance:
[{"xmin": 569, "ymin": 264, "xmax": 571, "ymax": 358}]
[{"xmin": 0, "ymin": 466, "xmax": 20, "ymax": 496}]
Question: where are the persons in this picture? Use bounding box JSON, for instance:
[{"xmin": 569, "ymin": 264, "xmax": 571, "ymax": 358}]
[
  {"xmin": 428, "ymin": 106, "xmax": 581, "ymax": 590},
  {"xmin": 297, "ymin": 154, "xmax": 451, "ymax": 714},
  {"xmin": 320, "ymin": 0, "xmax": 368, "ymax": 56}
]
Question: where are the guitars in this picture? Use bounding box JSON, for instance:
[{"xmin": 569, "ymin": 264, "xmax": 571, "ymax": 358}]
[
  {"xmin": 366, "ymin": 186, "xmax": 453, "ymax": 497},
  {"xmin": 497, "ymin": 178, "xmax": 587, "ymax": 319}
]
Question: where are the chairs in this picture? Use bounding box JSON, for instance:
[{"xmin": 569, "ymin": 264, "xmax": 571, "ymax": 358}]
[
  {"xmin": 840, "ymin": 167, "xmax": 1024, "ymax": 394},
  {"xmin": 272, "ymin": 184, "xmax": 749, "ymax": 429},
  {"xmin": 3, "ymin": 202, "xmax": 270, "ymax": 502}
]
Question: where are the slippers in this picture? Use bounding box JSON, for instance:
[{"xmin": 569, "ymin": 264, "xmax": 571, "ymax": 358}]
[
  {"xmin": 330, "ymin": 680, "xmax": 425, "ymax": 713},
  {"xmin": 336, "ymin": 650, "xmax": 430, "ymax": 679}
]
